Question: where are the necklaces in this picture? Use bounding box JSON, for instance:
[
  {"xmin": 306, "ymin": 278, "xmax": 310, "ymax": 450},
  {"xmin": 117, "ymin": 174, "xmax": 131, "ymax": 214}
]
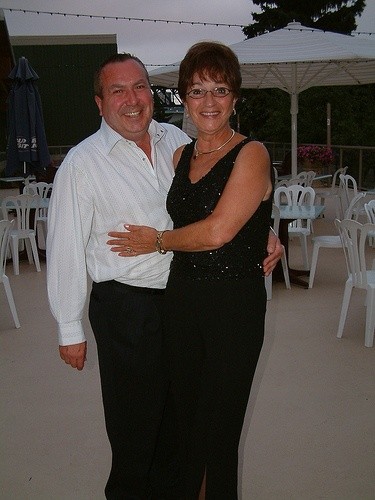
[{"xmin": 194, "ymin": 128, "xmax": 235, "ymax": 160}]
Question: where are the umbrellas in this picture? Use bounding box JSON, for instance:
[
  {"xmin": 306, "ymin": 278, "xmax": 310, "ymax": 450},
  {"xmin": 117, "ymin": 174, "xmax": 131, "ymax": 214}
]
[
  {"xmin": 5, "ymin": 57, "xmax": 49, "ymax": 180},
  {"xmin": 147, "ymin": 20, "xmax": 375, "ymax": 187}
]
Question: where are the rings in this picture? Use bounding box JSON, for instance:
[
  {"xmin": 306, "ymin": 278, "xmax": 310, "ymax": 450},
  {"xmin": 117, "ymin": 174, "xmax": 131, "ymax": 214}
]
[{"xmin": 126, "ymin": 246, "xmax": 132, "ymax": 252}]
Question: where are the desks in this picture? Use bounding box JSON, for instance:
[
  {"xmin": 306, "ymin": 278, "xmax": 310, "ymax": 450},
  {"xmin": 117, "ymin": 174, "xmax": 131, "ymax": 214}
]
[
  {"xmin": 0, "ymin": 175, "xmax": 36, "ymax": 193},
  {"xmin": 271, "ymin": 204, "xmax": 326, "ymax": 291},
  {"xmin": 275, "ymin": 173, "xmax": 332, "ymax": 219},
  {"xmin": 0, "ymin": 197, "xmax": 50, "ymax": 265}
]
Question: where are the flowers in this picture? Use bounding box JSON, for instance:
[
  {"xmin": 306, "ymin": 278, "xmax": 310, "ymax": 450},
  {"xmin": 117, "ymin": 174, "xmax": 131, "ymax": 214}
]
[{"xmin": 296, "ymin": 145, "xmax": 335, "ymax": 166}]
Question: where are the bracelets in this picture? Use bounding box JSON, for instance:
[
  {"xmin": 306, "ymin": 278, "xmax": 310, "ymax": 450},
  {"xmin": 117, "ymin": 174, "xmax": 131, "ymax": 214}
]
[{"xmin": 154, "ymin": 230, "xmax": 170, "ymax": 255}]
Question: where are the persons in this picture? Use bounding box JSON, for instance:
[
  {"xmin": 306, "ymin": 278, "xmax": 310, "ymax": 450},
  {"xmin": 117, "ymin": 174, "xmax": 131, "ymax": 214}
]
[
  {"xmin": 107, "ymin": 41, "xmax": 274, "ymax": 500},
  {"xmin": 45, "ymin": 51, "xmax": 285, "ymax": 500}
]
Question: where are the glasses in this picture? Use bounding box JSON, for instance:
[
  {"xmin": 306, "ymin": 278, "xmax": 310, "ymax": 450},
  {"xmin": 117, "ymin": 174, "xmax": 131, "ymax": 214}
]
[{"xmin": 186, "ymin": 86, "xmax": 233, "ymax": 99}]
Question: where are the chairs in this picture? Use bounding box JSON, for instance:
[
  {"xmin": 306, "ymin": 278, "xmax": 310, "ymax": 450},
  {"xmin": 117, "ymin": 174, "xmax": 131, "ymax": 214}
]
[
  {"xmin": 1, "ymin": 194, "xmax": 43, "ymax": 276},
  {"xmin": 0, "ymin": 218, "xmax": 21, "ymax": 328},
  {"xmin": 264, "ymin": 167, "xmax": 375, "ymax": 347},
  {"xmin": 22, "ymin": 181, "xmax": 54, "ymax": 265}
]
